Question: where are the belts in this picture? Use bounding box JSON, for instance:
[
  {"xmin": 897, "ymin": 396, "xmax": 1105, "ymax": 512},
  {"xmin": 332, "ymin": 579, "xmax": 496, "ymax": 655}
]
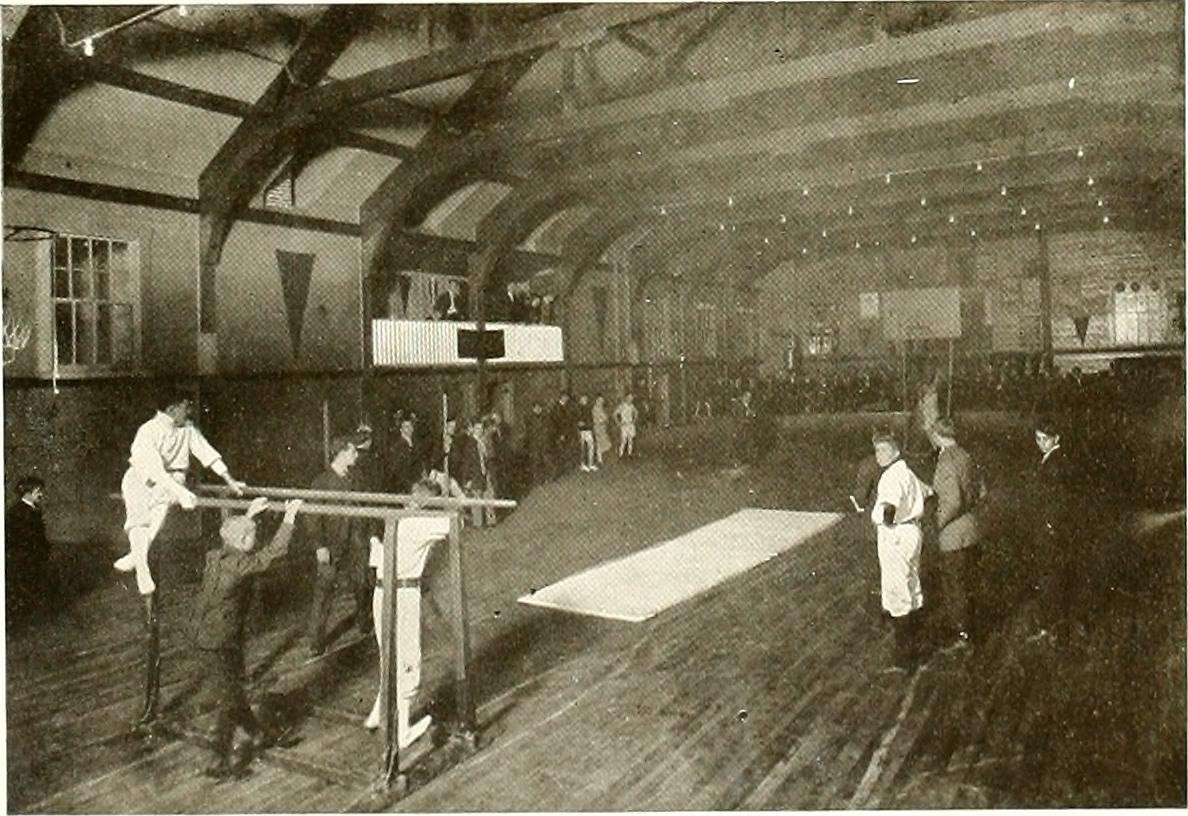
[{"xmin": 375, "ymin": 578, "xmax": 421, "ymax": 588}]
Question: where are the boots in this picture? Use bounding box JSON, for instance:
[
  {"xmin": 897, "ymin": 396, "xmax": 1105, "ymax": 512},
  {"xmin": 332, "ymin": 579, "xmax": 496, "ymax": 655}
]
[
  {"xmin": 1024, "ymin": 621, "xmax": 1061, "ymax": 651},
  {"xmin": 397, "ymin": 701, "xmax": 432, "ymax": 749},
  {"xmin": 939, "ymin": 629, "xmax": 975, "ymax": 659},
  {"xmin": 889, "ymin": 613, "xmax": 916, "ymax": 668},
  {"xmin": 236, "ymin": 704, "xmax": 294, "ymax": 749},
  {"xmin": 366, "ymin": 693, "xmax": 380, "ymax": 729},
  {"xmin": 130, "ymin": 527, "xmax": 156, "ymax": 595},
  {"xmin": 114, "ymin": 522, "xmax": 159, "ymax": 572},
  {"xmin": 208, "ymin": 708, "xmax": 246, "ymax": 778}
]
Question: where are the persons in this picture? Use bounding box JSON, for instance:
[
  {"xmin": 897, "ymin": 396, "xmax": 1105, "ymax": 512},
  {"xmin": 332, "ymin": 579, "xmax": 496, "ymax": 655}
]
[
  {"xmin": 929, "ymin": 417, "xmax": 983, "ymax": 655},
  {"xmin": 508, "ymin": 282, "xmax": 555, "ymax": 325},
  {"xmin": 185, "ymin": 498, "xmax": 303, "ymax": 781},
  {"xmin": 363, "ymin": 471, "xmax": 467, "ymax": 750},
  {"xmin": 871, "ymin": 429, "xmax": 933, "ymax": 672},
  {"xmin": 5, "ymin": 478, "xmax": 53, "ymax": 625},
  {"xmin": 697, "ymin": 346, "xmax": 1186, "ymax": 464},
  {"xmin": 307, "ymin": 433, "xmax": 373, "ymax": 657},
  {"xmin": 112, "ymin": 386, "xmax": 247, "ymax": 595},
  {"xmin": 356, "ymin": 411, "xmax": 511, "ymax": 529},
  {"xmin": 1028, "ymin": 419, "xmax": 1077, "ymax": 646},
  {"xmin": 851, "ymin": 426, "xmax": 892, "ymax": 593},
  {"xmin": 613, "ymin": 393, "xmax": 638, "ymax": 458},
  {"xmin": 526, "ymin": 390, "xmax": 612, "ymax": 483},
  {"xmin": 433, "ymin": 279, "xmax": 465, "ymax": 320}
]
